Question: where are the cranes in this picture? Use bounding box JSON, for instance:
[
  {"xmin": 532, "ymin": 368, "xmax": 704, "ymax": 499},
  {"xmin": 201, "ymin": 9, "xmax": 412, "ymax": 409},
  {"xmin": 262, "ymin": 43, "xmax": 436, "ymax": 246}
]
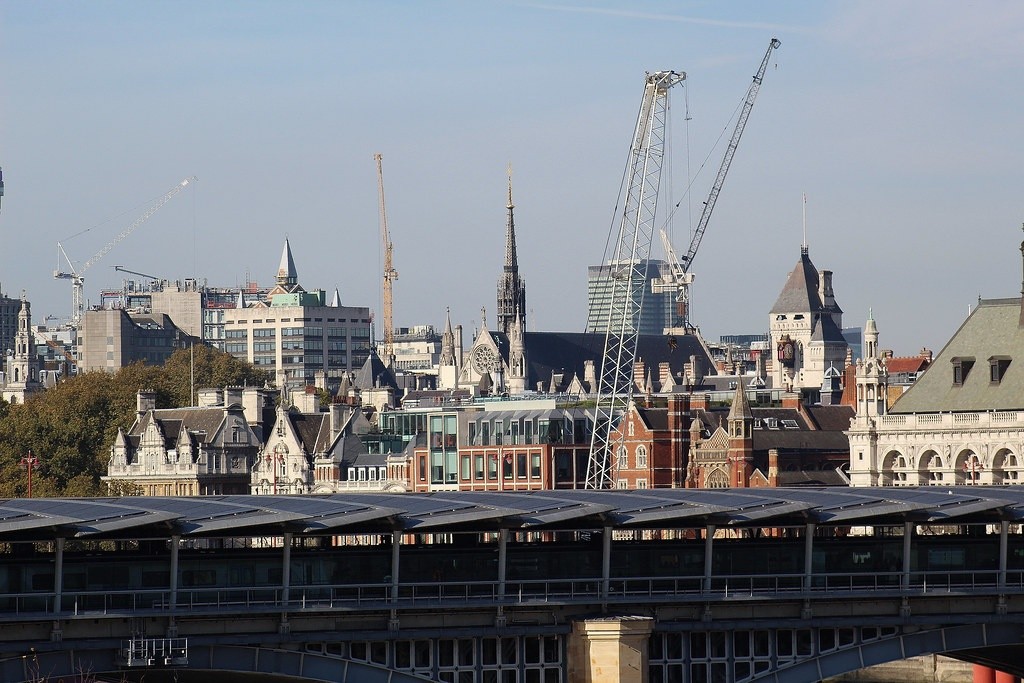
[
  {"xmin": 53, "ymin": 176, "xmax": 198, "ymax": 327},
  {"xmin": 658, "ymin": 36, "xmax": 785, "ymax": 303},
  {"xmin": 373, "ymin": 152, "xmax": 398, "ymax": 363}
]
